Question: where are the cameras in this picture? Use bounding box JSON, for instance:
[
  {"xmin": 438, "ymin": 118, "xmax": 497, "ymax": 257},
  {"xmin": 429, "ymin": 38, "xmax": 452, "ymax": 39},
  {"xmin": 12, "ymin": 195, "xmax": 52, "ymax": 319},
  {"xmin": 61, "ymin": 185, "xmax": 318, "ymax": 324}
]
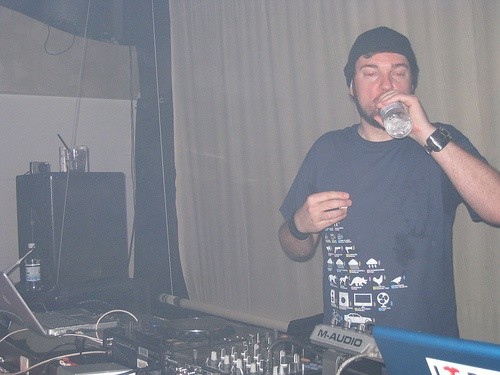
[{"xmin": 30, "ymin": 163, "xmax": 51, "ymax": 174}]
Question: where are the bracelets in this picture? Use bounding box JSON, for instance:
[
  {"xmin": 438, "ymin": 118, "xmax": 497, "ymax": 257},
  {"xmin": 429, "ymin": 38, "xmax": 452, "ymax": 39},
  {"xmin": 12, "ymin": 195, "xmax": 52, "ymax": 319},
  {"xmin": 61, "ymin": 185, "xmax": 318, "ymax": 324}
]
[
  {"xmin": 423, "ymin": 127, "xmax": 454, "ymax": 156},
  {"xmin": 287, "ymin": 212, "xmax": 312, "ymax": 241}
]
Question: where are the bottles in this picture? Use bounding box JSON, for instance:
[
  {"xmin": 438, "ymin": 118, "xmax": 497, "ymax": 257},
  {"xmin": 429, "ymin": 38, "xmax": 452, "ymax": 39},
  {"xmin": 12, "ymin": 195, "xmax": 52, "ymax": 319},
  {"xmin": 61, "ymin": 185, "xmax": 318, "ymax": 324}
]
[
  {"xmin": 23, "ymin": 243, "xmax": 43, "ymax": 292},
  {"xmin": 59, "ymin": 144, "xmax": 89, "ymax": 172},
  {"xmin": 379, "ymin": 101, "xmax": 413, "ymax": 139}
]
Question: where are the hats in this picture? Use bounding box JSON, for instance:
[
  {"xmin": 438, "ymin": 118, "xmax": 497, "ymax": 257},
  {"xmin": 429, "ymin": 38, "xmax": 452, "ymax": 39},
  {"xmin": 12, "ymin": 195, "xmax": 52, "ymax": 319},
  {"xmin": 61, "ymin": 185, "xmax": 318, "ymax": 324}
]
[{"xmin": 344, "ymin": 25, "xmax": 419, "ymax": 76}]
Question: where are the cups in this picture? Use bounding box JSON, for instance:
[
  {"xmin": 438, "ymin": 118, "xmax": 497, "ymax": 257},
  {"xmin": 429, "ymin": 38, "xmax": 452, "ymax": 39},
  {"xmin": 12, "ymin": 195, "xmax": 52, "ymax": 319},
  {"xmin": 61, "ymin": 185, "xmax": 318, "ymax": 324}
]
[
  {"xmin": 30, "ymin": 161, "xmax": 50, "ymax": 175},
  {"xmin": 65, "ymin": 149, "xmax": 86, "ymax": 172}
]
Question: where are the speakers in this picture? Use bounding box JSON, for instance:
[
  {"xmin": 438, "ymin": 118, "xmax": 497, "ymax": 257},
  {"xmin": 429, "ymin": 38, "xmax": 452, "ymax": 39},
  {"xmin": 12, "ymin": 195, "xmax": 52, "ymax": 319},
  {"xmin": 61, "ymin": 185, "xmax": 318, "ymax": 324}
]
[{"xmin": 15, "ymin": 172, "xmax": 129, "ymax": 323}]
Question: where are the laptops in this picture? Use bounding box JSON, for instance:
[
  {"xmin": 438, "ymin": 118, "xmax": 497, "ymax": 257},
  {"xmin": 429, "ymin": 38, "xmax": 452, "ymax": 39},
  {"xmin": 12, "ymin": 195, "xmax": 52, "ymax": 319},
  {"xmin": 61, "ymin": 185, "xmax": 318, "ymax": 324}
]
[
  {"xmin": 373, "ymin": 325, "xmax": 500, "ymax": 375},
  {"xmin": 0, "ymin": 271, "xmax": 118, "ymax": 337}
]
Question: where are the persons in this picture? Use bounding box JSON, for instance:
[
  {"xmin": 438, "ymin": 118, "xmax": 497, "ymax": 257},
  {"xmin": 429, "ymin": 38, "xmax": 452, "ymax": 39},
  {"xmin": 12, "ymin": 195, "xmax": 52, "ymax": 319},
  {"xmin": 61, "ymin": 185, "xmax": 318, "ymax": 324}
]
[{"xmin": 277, "ymin": 26, "xmax": 500, "ymax": 338}]
